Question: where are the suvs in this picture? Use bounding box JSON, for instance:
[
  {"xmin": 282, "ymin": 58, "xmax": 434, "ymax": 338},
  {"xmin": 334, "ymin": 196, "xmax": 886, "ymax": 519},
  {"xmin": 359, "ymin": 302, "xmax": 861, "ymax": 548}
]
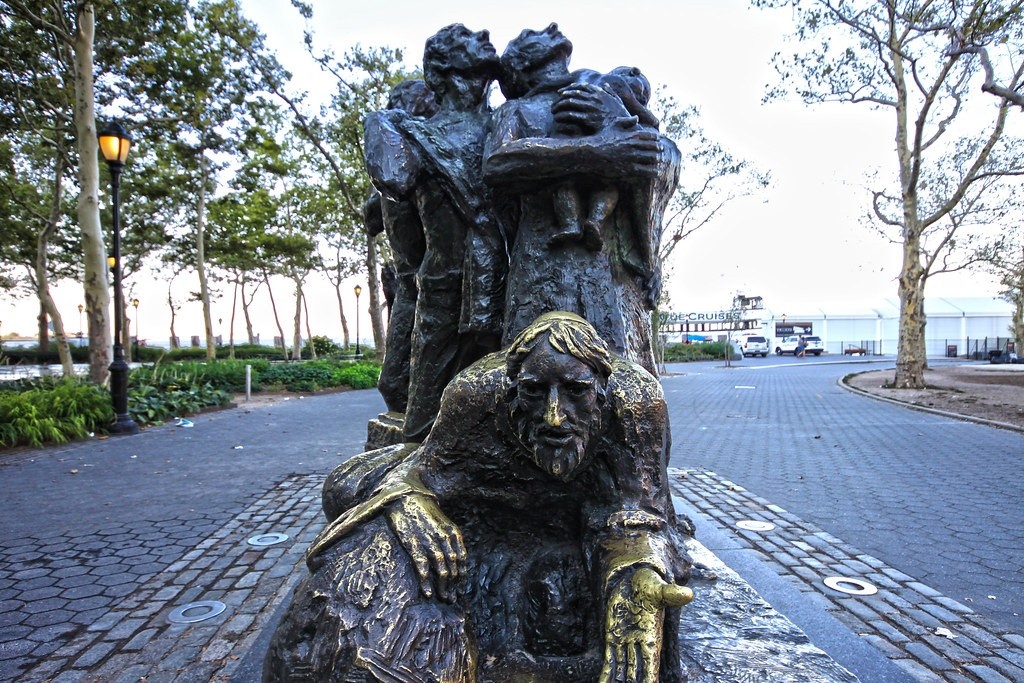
[
  {"xmin": 738, "ymin": 335, "xmax": 768, "ymax": 357},
  {"xmin": 774, "ymin": 335, "xmax": 824, "ymax": 358}
]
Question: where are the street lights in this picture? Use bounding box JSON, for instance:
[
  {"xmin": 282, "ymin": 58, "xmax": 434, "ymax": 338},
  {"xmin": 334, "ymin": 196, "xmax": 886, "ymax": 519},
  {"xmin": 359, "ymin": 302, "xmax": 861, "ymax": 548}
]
[
  {"xmin": 77, "ymin": 304, "xmax": 83, "ymax": 348},
  {"xmin": 97, "ymin": 114, "xmax": 138, "ymax": 435},
  {"xmin": 781, "ymin": 313, "xmax": 787, "ymax": 343},
  {"xmin": 133, "ymin": 299, "xmax": 141, "ymax": 362},
  {"xmin": 353, "ymin": 285, "xmax": 362, "ymax": 360}
]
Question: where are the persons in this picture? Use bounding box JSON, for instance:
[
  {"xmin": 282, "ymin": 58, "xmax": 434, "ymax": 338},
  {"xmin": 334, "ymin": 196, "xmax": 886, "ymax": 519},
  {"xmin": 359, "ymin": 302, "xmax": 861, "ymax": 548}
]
[{"xmin": 266, "ymin": 26, "xmax": 696, "ymax": 683}]
